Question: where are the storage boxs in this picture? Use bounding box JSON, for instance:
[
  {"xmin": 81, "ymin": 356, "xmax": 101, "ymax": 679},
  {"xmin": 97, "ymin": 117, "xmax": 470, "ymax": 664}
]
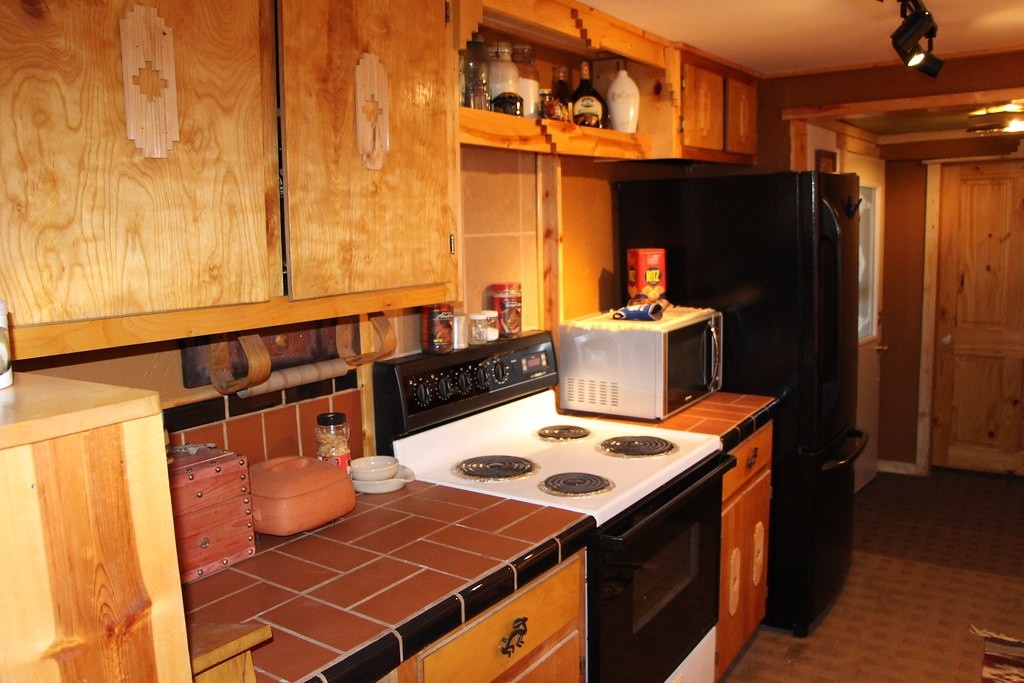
[
  {"xmin": 627, "ymin": 248, "xmax": 666, "ymax": 308},
  {"xmin": 166, "ymin": 445, "xmax": 256, "ymax": 582}
]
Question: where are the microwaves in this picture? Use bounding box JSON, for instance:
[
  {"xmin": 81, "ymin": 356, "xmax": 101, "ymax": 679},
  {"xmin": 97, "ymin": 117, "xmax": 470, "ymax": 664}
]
[{"xmin": 558, "ymin": 303, "xmax": 724, "ymax": 421}]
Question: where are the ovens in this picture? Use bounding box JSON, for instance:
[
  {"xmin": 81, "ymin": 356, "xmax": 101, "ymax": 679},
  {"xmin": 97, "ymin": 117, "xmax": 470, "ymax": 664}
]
[{"xmin": 585, "ymin": 452, "xmax": 737, "ymax": 683}]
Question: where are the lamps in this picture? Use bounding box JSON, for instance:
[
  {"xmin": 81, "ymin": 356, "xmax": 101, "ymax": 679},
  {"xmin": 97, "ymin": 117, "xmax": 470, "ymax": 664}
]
[{"xmin": 889, "ymin": 0, "xmax": 943, "ymax": 79}]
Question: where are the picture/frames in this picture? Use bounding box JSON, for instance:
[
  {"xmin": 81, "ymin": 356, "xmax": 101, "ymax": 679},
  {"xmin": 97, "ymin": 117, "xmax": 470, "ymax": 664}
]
[{"xmin": 815, "ymin": 149, "xmax": 836, "ymax": 172}]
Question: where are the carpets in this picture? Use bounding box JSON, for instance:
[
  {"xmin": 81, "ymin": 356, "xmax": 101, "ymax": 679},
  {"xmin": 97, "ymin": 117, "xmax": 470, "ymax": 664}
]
[{"xmin": 968, "ymin": 625, "xmax": 1024, "ymax": 683}]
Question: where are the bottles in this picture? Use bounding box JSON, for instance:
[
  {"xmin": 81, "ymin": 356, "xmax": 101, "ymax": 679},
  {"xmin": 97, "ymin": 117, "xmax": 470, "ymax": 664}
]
[
  {"xmin": 458, "ymin": 33, "xmax": 639, "ymax": 134},
  {"xmin": 420, "ymin": 302, "xmax": 455, "ymax": 352},
  {"xmin": 315, "ymin": 412, "xmax": 352, "ymax": 479},
  {"xmin": 490, "ymin": 282, "xmax": 522, "ymax": 339},
  {"xmin": 468, "ymin": 315, "xmax": 488, "ymax": 344},
  {"xmin": 480, "ymin": 310, "xmax": 499, "ymax": 341}
]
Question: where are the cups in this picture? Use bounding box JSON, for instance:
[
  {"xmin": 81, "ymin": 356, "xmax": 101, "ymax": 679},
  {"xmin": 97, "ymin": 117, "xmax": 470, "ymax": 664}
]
[{"xmin": 0, "ymin": 299, "xmax": 14, "ymax": 389}]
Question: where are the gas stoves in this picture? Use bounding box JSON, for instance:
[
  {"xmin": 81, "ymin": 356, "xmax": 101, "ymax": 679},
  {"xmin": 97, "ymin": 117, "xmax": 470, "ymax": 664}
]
[{"xmin": 373, "ymin": 329, "xmax": 721, "ymax": 528}]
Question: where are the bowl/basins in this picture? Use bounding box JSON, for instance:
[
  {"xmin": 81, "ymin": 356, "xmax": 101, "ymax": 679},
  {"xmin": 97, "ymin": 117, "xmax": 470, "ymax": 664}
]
[{"xmin": 351, "ymin": 455, "xmax": 398, "ymax": 480}]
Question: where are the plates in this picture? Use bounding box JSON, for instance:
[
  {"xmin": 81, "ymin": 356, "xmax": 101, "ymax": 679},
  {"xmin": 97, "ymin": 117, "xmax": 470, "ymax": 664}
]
[{"xmin": 351, "ymin": 465, "xmax": 415, "ymax": 494}]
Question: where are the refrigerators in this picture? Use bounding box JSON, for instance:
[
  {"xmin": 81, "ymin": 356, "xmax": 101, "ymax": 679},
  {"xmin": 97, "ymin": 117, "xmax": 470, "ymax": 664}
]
[{"xmin": 612, "ymin": 170, "xmax": 868, "ymax": 637}]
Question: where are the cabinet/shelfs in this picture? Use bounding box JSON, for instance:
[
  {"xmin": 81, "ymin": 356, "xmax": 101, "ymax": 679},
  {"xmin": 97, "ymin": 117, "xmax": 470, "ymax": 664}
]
[
  {"xmin": 0, "ymin": 372, "xmax": 272, "ymax": 683},
  {"xmin": 715, "ymin": 416, "xmax": 774, "ymax": 683},
  {"xmin": 377, "ymin": 545, "xmax": 588, "ymax": 683},
  {"xmin": 0, "ymin": 0, "xmax": 766, "ymax": 364}
]
